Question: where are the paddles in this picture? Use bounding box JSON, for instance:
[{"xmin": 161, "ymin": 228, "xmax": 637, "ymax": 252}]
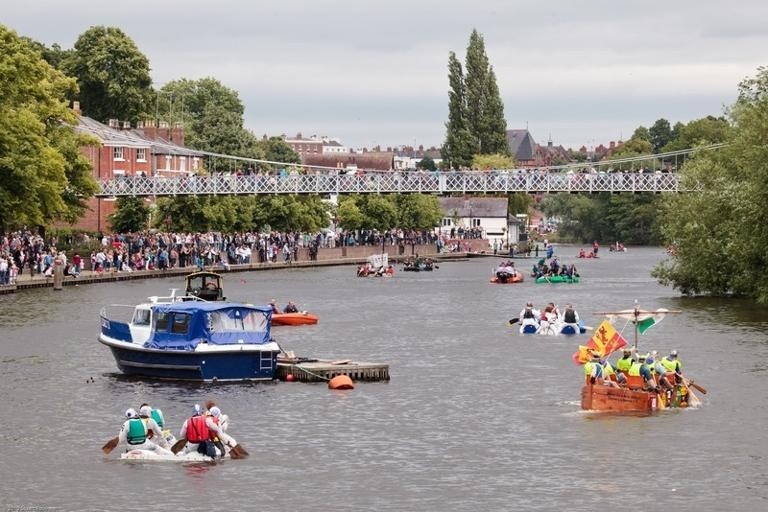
[
  {"xmin": 228, "ymin": 444, "xmax": 249, "ymax": 459},
  {"xmin": 510, "ymin": 318, "xmax": 520, "ymax": 324},
  {"xmin": 102, "ymin": 436, "xmax": 119, "ymax": 454},
  {"xmin": 652, "ymin": 371, "xmax": 665, "ymax": 410},
  {"xmin": 171, "ymin": 438, "xmax": 187, "ymax": 454},
  {"xmin": 664, "ymin": 365, "xmax": 707, "ymax": 402}
]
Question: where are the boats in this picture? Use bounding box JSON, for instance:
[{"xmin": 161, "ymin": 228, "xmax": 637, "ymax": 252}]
[
  {"xmin": 609, "ymin": 247, "xmax": 627, "ymax": 254},
  {"xmin": 519, "ymin": 316, "xmax": 585, "ymax": 336},
  {"xmin": 120, "ymin": 430, "xmax": 237, "ymax": 464},
  {"xmin": 577, "ymin": 255, "xmax": 600, "ymax": 259},
  {"xmin": 271, "ymin": 313, "xmax": 319, "ymax": 326},
  {"xmin": 97, "ymin": 287, "xmax": 282, "ymax": 386},
  {"xmin": 536, "ymin": 271, "xmax": 581, "ymax": 283},
  {"xmin": 580, "ymin": 297, "xmax": 683, "ymax": 413},
  {"xmin": 362, "ymin": 269, "xmax": 392, "ymax": 277},
  {"xmin": 491, "ymin": 271, "xmax": 523, "ymax": 282},
  {"xmin": 182, "ymin": 272, "xmax": 226, "ymax": 304},
  {"xmin": 403, "ymin": 266, "xmax": 433, "ymax": 272}
]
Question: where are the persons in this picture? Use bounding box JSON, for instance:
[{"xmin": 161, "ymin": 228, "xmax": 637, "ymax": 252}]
[
  {"xmin": 95, "ymin": 161, "xmax": 685, "ymax": 191},
  {"xmin": 518, "ymin": 301, "xmax": 581, "ymax": 337},
  {"xmin": 335, "ymin": 226, "xmax": 484, "ymax": 254},
  {"xmin": 140, "ymin": 403, "xmax": 177, "ymax": 445},
  {"xmin": 90, "ymin": 228, "xmax": 257, "ymax": 273},
  {"xmin": 254, "ymin": 229, "xmax": 336, "ymax": 263},
  {"xmin": 119, "ymin": 408, "xmax": 175, "ymax": 457},
  {"xmin": 579, "ymin": 347, "xmax": 684, "ymax": 393},
  {"xmin": 1, "ymin": 223, "xmax": 86, "ymax": 290},
  {"xmin": 139, "ymin": 406, "xmax": 169, "ymax": 450},
  {"xmin": 179, "ymin": 399, "xmax": 232, "ymax": 458},
  {"xmin": 267, "ymin": 298, "xmax": 283, "ymax": 314},
  {"xmin": 357, "ymin": 253, "xmax": 441, "ymax": 278},
  {"xmin": 283, "ymin": 300, "xmax": 298, "ymax": 314},
  {"xmin": 490, "ymin": 218, "xmax": 625, "ymax": 278}
]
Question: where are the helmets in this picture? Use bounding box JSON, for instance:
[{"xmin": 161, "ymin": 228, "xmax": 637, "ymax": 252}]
[
  {"xmin": 192, "ymin": 403, "xmax": 204, "ymax": 416},
  {"xmin": 138, "ymin": 405, "xmax": 152, "ymax": 417},
  {"xmin": 585, "ymin": 348, "xmax": 678, "ymax": 364},
  {"xmin": 207, "ymin": 407, "xmax": 221, "ymax": 417},
  {"xmin": 125, "ymin": 408, "xmax": 137, "ymax": 419},
  {"xmin": 526, "ymin": 301, "xmax": 574, "ymax": 309}
]
